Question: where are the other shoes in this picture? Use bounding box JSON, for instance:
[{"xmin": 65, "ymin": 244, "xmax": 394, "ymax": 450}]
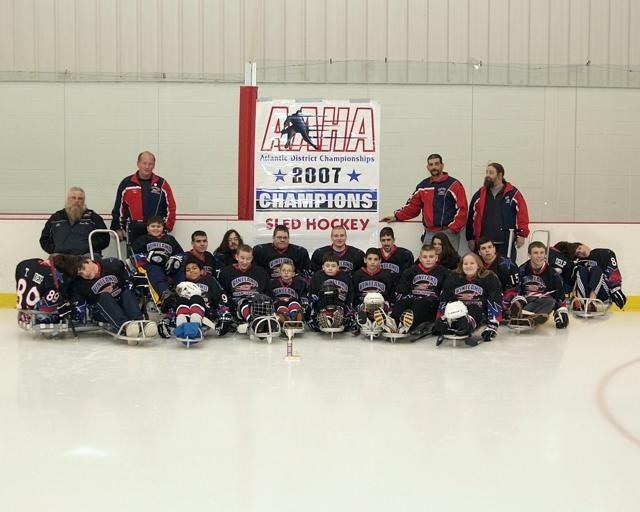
[
  {"xmin": 177, "ymin": 315, "xmax": 188, "ymax": 329},
  {"xmin": 509, "ymin": 300, "xmax": 521, "ymax": 324},
  {"xmin": 137, "ymin": 321, "xmax": 158, "ymax": 345},
  {"xmin": 524, "ymin": 313, "xmax": 548, "ymax": 327},
  {"xmin": 126, "ymin": 323, "xmax": 139, "ymax": 345},
  {"xmin": 279, "ymin": 306, "xmax": 414, "ymax": 334},
  {"xmin": 191, "ymin": 313, "xmax": 201, "ymax": 326}
]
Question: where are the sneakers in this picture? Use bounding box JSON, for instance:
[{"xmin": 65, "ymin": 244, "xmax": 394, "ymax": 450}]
[
  {"xmin": 588, "ymin": 302, "xmax": 597, "ymax": 313},
  {"xmin": 571, "ymin": 296, "xmax": 582, "ymax": 311}
]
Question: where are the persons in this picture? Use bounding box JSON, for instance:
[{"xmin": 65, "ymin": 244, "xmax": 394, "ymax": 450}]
[
  {"xmin": 110, "ymin": 152, "xmax": 175, "ymax": 258},
  {"xmin": 40, "ymin": 186, "xmax": 109, "ymax": 262},
  {"xmin": 379, "ymin": 154, "xmax": 468, "ymax": 254},
  {"xmin": 465, "ymin": 162, "xmax": 530, "ymax": 264},
  {"xmin": 69, "ymin": 217, "xmax": 627, "ymax": 346}
]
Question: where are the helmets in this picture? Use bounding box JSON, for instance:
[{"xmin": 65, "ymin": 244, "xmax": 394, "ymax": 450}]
[
  {"xmin": 176, "ymin": 281, "xmax": 201, "ymax": 300},
  {"xmin": 444, "ymin": 300, "xmax": 468, "ymax": 320},
  {"xmin": 364, "ymin": 292, "xmax": 385, "ymax": 308},
  {"xmin": 321, "ymin": 283, "xmax": 338, "ymax": 303},
  {"xmin": 250, "ymin": 294, "xmax": 273, "ymax": 314}
]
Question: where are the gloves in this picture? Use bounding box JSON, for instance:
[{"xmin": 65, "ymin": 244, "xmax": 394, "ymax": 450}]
[
  {"xmin": 610, "ymin": 286, "xmax": 626, "ymax": 309},
  {"xmin": 147, "ymin": 250, "xmax": 166, "ymax": 266},
  {"xmin": 553, "ymin": 310, "xmax": 569, "ymax": 328},
  {"xmin": 165, "ymin": 255, "xmax": 183, "ymax": 276}
]
